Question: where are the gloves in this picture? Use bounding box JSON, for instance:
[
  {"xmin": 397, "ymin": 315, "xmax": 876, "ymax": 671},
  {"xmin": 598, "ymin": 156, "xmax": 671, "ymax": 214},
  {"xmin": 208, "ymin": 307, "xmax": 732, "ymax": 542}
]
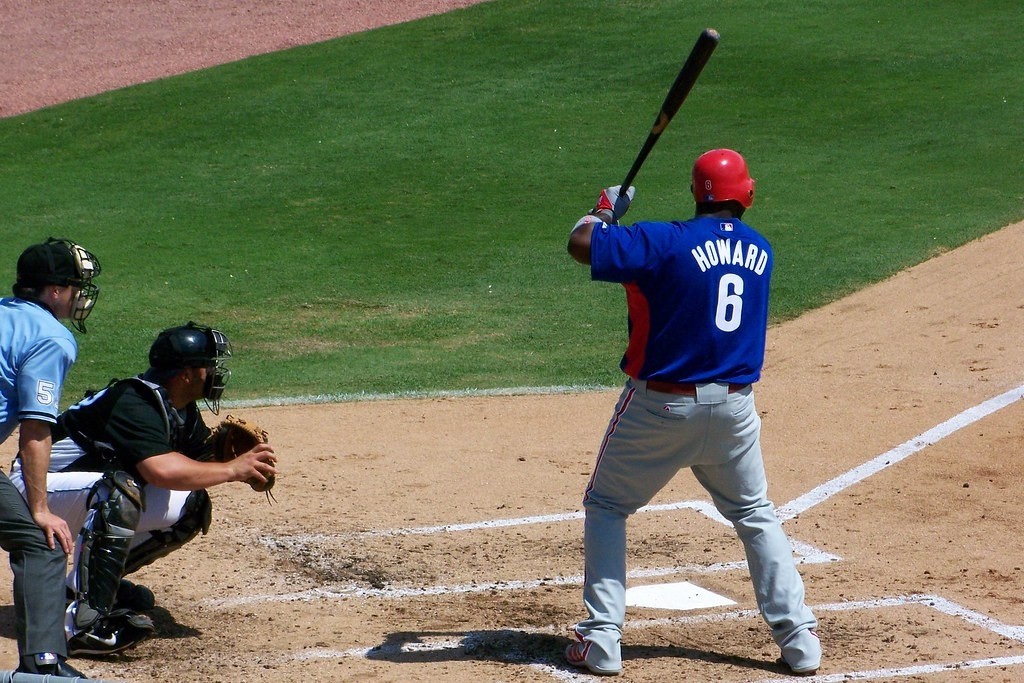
[
  {"xmin": 594, "ymin": 184, "xmax": 636, "ymax": 223},
  {"xmin": 588, "ymin": 207, "xmax": 621, "ymax": 226}
]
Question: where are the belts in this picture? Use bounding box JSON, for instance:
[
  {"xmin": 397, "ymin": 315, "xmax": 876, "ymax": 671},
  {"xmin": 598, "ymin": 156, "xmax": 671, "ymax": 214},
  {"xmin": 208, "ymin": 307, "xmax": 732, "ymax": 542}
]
[{"xmin": 646, "ymin": 379, "xmax": 742, "ymax": 396}]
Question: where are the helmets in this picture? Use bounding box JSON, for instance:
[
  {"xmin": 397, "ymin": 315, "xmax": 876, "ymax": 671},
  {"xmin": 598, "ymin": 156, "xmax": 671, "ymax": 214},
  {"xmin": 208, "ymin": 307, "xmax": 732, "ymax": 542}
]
[
  {"xmin": 142, "ymin": 325, "xmax": 215, "ymax": 382},
  {"xmin": 690, "ymin": 149, "xmax": 755, "ymax": 208}
]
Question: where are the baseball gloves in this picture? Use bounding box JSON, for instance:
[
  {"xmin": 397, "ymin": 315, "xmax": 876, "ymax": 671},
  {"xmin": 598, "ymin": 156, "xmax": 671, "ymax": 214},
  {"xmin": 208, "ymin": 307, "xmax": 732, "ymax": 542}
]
[{"xmin": 201, "ymin": 416, "xmax": 276, "ymax": 493}]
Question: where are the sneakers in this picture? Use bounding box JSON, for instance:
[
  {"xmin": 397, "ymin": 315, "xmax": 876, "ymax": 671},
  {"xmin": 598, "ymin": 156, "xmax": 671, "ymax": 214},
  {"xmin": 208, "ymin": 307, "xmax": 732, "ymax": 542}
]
[
  {"xmin": 68, "ymin": 619, "xmax": 149, "ymax": 656},
  {"xmin": 780, "ymin": 630, "xmax": 821, "ymax": 673},
  {"xmin": 65, "ymin": 585, "xmax": 150, "ymax": 614},
  {"xmin": 564, "ymin": 642, "xmax": 618, "ymax": 676}
]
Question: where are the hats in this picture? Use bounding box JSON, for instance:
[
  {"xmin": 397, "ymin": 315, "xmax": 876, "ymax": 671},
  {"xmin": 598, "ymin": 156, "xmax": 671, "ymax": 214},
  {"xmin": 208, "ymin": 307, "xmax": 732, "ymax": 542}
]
[{"xmin": 17, "ymin": 243, "xmax": 85, "ymax": 286}]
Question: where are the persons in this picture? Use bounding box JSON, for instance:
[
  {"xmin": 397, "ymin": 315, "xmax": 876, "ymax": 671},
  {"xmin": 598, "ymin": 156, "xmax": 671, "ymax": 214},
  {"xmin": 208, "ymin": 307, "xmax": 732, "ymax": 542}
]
[
  {"xmin": 0, "ymin": 237, "xmax": 101, "ymax": 683},
  {"xmin": 564, "ymin": 148, "xmax": 822, "ymax": 675},
  {"xmin": 8, "ymin": 321, "xmax": 279, "ymax": 658}
]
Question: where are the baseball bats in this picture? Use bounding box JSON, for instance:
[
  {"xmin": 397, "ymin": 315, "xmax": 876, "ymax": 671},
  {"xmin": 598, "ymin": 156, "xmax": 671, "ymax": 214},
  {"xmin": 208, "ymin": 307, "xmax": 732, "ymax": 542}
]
[{"xmin": 618, "ymin": 27, "xmax": 721, "ymax": 198}]
